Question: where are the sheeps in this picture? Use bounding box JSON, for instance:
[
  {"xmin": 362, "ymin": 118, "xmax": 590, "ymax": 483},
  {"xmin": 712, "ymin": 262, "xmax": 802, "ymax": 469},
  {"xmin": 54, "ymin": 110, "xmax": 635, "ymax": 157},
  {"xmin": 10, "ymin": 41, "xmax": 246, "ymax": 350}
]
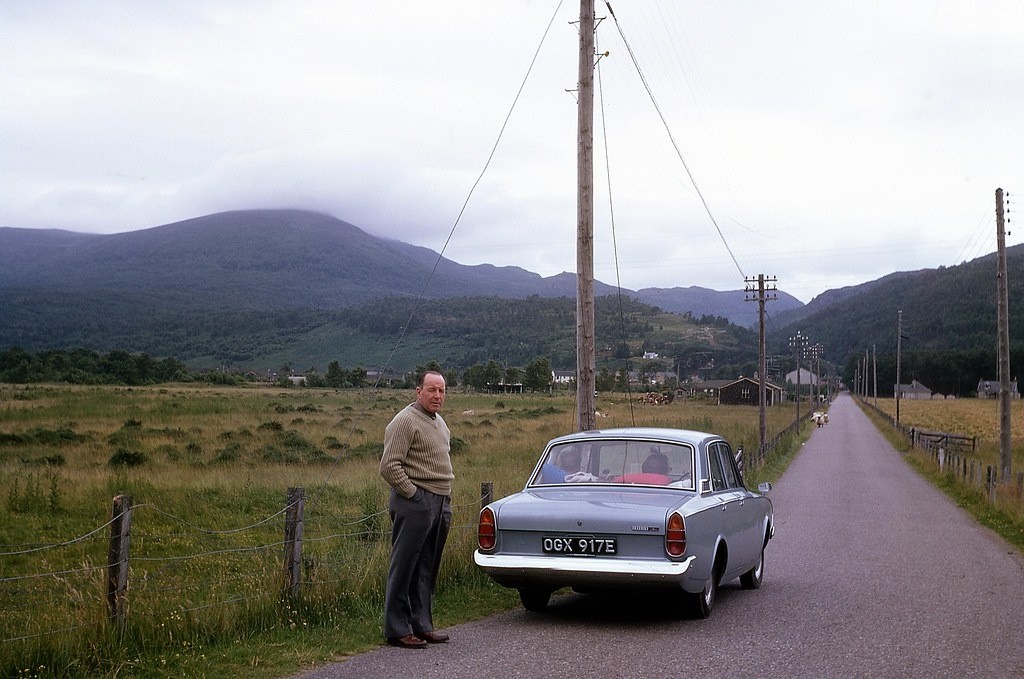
[
  {"xmin": 594, "ymin": 410, "xmax": 610, "ymax": 418},
  {"xmin": 461, "ymin": 409, "xmax": 475, "ymax": 416},
  {"xmin": 810, "ymin": 411, "xmax": 830, "ymax": 428}
]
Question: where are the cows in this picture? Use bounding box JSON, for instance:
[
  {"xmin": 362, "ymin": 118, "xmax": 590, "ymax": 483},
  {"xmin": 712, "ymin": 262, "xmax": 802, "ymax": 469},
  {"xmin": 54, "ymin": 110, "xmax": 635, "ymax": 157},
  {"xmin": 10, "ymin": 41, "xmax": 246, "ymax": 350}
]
[{"xmin": 638, "ymin": 391, "xmax": 666, "ymax": 407}]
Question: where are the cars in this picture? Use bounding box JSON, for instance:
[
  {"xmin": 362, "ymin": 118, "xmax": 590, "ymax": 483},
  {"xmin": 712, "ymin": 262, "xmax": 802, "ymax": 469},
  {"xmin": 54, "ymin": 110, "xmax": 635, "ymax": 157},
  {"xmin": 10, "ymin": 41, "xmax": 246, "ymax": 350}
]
[{"xmin": 474, "ymin": 427, "xmax": 775, "ymax": 618}]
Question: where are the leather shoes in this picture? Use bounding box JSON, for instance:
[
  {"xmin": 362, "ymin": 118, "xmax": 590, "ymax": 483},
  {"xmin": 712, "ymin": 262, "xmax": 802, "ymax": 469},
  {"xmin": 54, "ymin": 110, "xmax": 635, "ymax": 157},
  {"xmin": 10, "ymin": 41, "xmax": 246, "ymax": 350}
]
[
  {"xmin": 387, "ymin": 633, "xmax": 429, "ymax": 648},
  {"xmin": 414, "ymin": 629, "xmax": 449, "ymax": 643}
]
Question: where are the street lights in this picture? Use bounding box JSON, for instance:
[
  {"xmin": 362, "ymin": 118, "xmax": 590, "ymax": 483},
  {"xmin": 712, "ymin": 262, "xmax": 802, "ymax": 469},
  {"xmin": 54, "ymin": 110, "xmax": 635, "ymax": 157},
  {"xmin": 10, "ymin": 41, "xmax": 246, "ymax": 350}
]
[
  {"xmin": 743, "ymin": 273, "xmax": 779, "ymax": 467},
  {"xmin": 789, "ymin": 331, "xmax": 824, "ymax": 437}
]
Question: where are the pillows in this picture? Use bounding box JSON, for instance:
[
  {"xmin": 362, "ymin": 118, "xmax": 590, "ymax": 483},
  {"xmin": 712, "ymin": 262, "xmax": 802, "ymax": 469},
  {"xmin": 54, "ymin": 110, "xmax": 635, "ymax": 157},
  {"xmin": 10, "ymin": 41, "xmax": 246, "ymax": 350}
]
[
  {"xmin": 612, "ymin": 472, "xmax": 673, "ymax": 485},
  {"xmin": 542, "ymin": 464, "xmax": 571, "ymax": 483}
]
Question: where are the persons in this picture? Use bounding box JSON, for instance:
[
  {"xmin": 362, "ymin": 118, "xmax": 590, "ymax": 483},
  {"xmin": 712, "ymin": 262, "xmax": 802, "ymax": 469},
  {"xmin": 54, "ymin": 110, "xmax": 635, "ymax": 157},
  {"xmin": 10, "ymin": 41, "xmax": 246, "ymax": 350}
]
[
  {"xmin": 380, "ymin": 371, "xmax": 455, "ymax": 648},
  {"xmin": 558, "ymin": 446, "xmax": 581, "ymax": 473},
  {"xmin": 641, "ymin": 453, "xmax": 672, "ymax": 474}
]
